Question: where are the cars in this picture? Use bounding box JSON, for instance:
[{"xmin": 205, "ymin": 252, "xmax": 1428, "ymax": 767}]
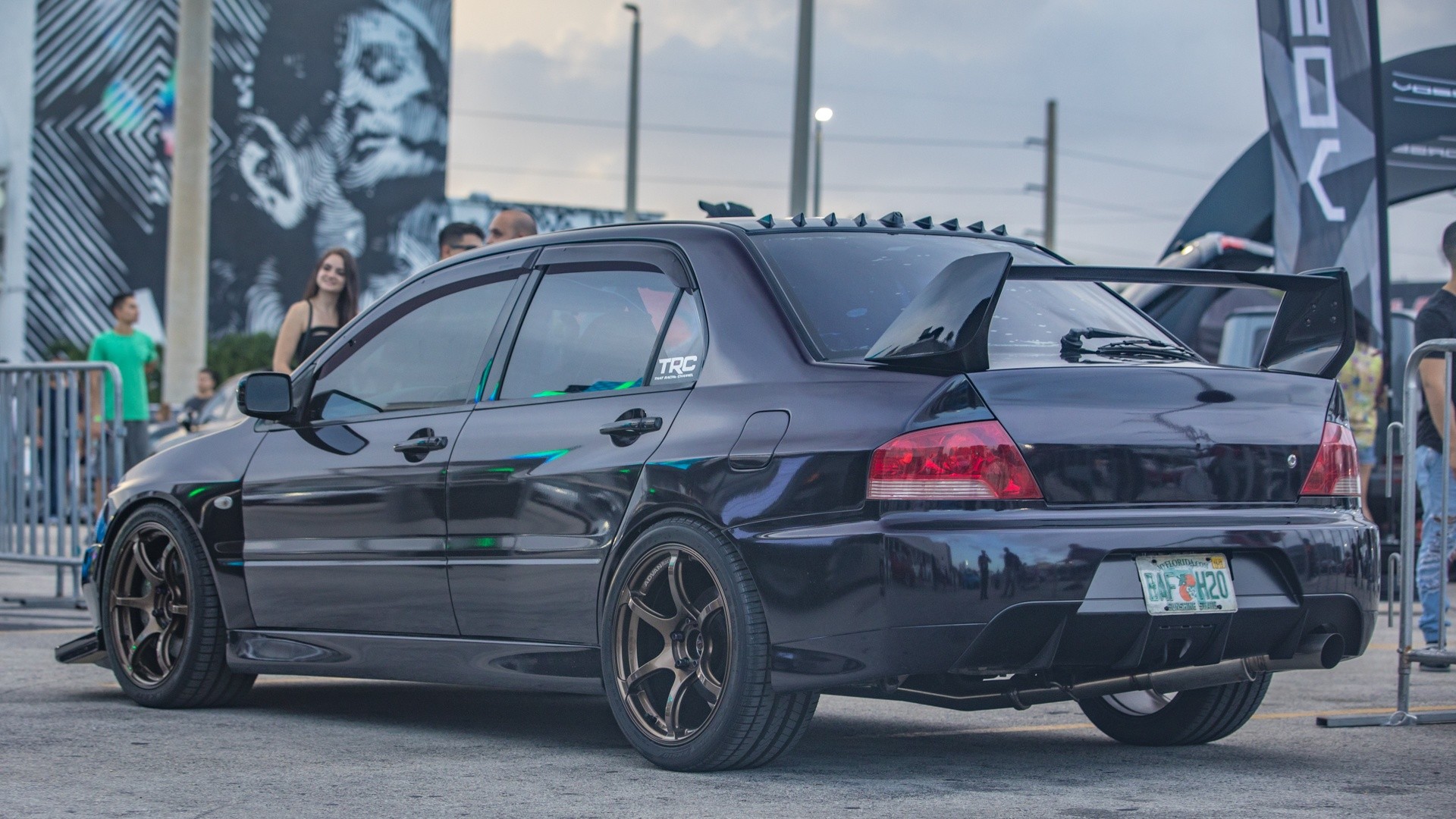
[
  {"xmin": 1113, "ymin": 227, "xmax": 1423, "ymax": 605},
  {"xmin": 146, "ymin": 370, "xmax": 252, "ymax": 456},
  {"xmin": 55, "ymin": 210, "xmax": 1382, "ymax": 773}
]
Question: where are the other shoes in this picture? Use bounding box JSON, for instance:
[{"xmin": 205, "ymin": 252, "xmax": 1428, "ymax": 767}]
[{"xmin": 1419, "ymin": 645, "xmax": 1450, "ymax": 671}]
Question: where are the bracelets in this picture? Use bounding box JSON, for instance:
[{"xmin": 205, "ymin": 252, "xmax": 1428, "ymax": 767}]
[{"xmin": 90, "ymin": 415, "xmax": 105, "ymax": 424}]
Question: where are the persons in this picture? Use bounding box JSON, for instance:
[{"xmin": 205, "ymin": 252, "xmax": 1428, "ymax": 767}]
[
  {"xmin": 1411, "ymin": 221, "xmax": 1456, "ymax": 675},
  {"xmin": 272, "ymin": 249, "xmax": 359, "ymax": 375},
  {"xmin": 0, "ymin": 291, "xmax": 222, "ymax": 527},
  {"xmin": 1336, "ymin": 308, "xmax": 1384, "ymax": 522},
  {"xmin": 438, "ymin": 206, "xmax": 537, "ymax": 264}
]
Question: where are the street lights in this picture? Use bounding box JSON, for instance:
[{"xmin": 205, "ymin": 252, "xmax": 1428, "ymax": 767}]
[
  {"xmin": 812, "ymin": 107, "xmax": 833, "ymax": 218},
  {"xmin": 621, "ymin": 3, "xmax": 642, "ymax": 222}
]
